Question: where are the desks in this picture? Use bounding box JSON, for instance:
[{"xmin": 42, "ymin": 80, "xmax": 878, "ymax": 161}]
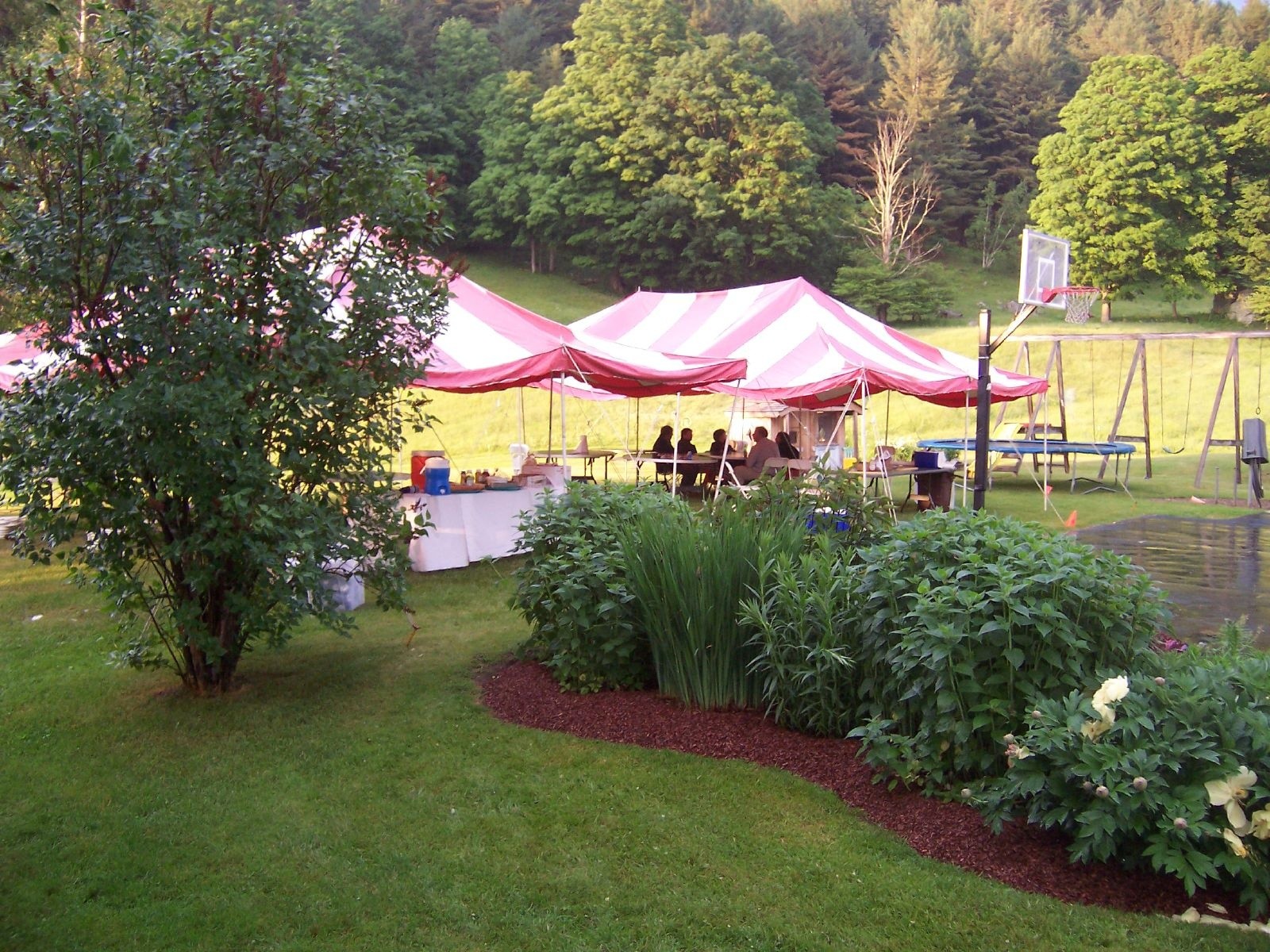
[
  {"xmin": 623, "ymin": 454, "xmax": 718, "ymax": 499},
  {"xmin": 700, "ymin": 453, "xmax": 743, "ymax": 460},
  {"xmin": 533, "ymin": 450, "xmax": 616, "ymax": 484},
  {"xmin": 849, "ymin": 468, "xmax": 952, "ymax": 514},
  {"xmin": 265, "ymin": 484, "xmax": 562, "ymax": 573}
]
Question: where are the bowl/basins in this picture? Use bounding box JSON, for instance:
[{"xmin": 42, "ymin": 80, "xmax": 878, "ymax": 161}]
[{"xmin": 853, "ymin": 462, "xmax": 869, "ymax": 471}]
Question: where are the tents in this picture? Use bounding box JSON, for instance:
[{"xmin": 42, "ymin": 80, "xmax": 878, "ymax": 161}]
[
  {"xmin": 0, "ymin": 212, "xmax": 748, "ymax": 526},
  {"xmin": 517, "ymin": 275, "xmax": 1048, "ymax": 525}
]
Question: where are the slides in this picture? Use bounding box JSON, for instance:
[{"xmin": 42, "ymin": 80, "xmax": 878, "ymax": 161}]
[{"xmin": 966, "ymin": 421, "xmax": 1023, "ymax": 479}]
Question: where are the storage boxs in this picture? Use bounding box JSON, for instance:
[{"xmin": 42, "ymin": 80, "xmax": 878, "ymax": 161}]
[
  {"xmin": 806, "ymin": 511, "xmax": 850, "ymax": 534},
  {"xmin": 307, "ymin": 559, "xmax": 366, "ymax": 612}
]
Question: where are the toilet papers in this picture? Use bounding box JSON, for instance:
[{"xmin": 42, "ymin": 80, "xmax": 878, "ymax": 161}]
[{"xmin": 577, "ymin": 435, "xmax": 588, "ymax": 453}]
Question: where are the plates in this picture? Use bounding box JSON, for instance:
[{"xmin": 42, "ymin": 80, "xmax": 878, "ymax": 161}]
[{"xmin": 450, "ymin": 484, "xmax": 485, "ymax": 492}]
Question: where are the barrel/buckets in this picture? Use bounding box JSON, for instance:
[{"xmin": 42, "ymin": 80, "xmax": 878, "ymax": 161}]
[
  {"xmin": 420, "ymin": 457, "xmax": 451, "ymax": 495},
  {"xmin": 917, "ymin": 471, "xmax": 954, "ymax": 513},
  {"xmin": 411, "ymin": 450, "xmax": 445, "ymax": 493}
]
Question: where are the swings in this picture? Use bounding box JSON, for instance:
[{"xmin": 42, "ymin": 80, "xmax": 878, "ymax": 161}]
[
  {"xmin": 1159, "ymin": 339, "xmax": 1194, "ymax": 454},
  {"xmin": 1089, "ymin": 341, "xmax": 1124, "ymax": 442}
]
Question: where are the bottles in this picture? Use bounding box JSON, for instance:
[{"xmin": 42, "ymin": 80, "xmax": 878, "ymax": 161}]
[
  {"xmin": 466, "ymin": 469, "xmax": 474, "ymax": 486},
  {"xmin": 483, "ymin": 468, "xmax": 490, "ymax": 483},
  {"xmin": 869, "ymin": 457, "xmax": 876, "ymax": 471},
  {"xmin": 475, "ymin": 469, "xmax": 483, "ymax": 484},
  {"xmin": 743, "ymin": 448, "xmax": 747, "ymax": 458},
  {"xmin": 687, "ymin": 452, "xmax": 692, "ymax": 460},
  {"xmin": 461, "ymin": 471, "xmax": 467, "ymax": 486}
]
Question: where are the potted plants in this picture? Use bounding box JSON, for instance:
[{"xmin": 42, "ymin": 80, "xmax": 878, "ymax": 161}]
[{"xmin": 917, "ymin": 448, "xmax": 959, "ymax": 513}]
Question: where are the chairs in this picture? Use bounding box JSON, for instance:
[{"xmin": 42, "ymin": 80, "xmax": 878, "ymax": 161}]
[
  {"xmin": 788, "ymin": 459, "xmax": 823, "ymax": 479},
  {"xmin": 761, "ymin": 457, "xmax": 789, "ymax": 469}
]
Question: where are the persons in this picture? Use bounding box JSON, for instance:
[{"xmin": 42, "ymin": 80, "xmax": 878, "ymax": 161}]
[
  {"xmin": 731, "ymin": 426, "xmax": 782, "ymax": 486},
  {"xmin": 651, "ymin": 426, "xmax": 803, "ymax": 493}
]
